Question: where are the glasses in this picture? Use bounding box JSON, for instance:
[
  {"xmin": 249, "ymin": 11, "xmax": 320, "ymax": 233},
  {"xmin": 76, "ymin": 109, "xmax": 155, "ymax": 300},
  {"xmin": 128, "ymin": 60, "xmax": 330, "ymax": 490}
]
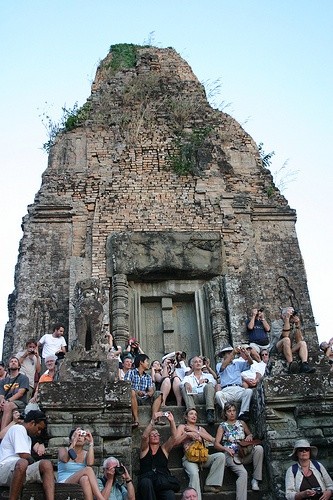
[
  {"xmin": 297, "ymin": 448, "xmax": 310, "ymax": 452},
  {"xmin": 261, "ymin": 354, "xmax": 267, "ymax": 356}
]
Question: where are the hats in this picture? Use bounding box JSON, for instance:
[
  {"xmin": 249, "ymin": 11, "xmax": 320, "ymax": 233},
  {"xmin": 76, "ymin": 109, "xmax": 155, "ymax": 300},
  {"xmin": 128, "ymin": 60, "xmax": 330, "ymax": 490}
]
[
  {"xmin": 288, "ymin": 439, "xmax": 317, "ymax": 461},
  {"xmin": 128, "ymin": 338, "xmax": 136, "ymax": 343},
  {"xmin": 218, "ymin": 347, "xmax": 234, "ymax": 358},
  {"xmin": 241, "ymin": 344, "xmax": 252, "ymax": 349}
]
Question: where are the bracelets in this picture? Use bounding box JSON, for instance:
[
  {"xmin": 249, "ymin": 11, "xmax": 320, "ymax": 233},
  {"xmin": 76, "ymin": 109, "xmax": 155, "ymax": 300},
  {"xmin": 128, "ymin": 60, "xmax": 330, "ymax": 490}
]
[
  {"xmin": 150, "ymin": 419, "xmax": 153, "ymax": 426},
  {"xmin": 197, "ymin": 430, "xmax": 200, "ymax": 434}
]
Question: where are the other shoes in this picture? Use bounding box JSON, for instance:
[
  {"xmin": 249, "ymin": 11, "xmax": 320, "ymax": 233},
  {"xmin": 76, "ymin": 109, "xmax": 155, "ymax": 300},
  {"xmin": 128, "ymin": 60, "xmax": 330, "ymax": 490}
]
[
  {"xmin": 132, "ymin": 421, "xmax": 139, "ymax": 427},
  {"xmin": 207, "ymin": 410, "xmax": 215, "ymax": 424},
  {"xmin": 155, "ymin": 421, "xmax": 166, "ymax": 425},
  {"xmin": 238, "ymin": 414, "xmax": 250, "ymax": 421},
  {"xmin": 203, "ymin": 485, "xmax": 220, "ymax": 493}
]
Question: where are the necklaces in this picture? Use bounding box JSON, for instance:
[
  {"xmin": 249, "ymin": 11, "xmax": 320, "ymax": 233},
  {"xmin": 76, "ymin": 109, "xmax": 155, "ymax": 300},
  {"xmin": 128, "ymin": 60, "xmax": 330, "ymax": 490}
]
[{"xmin": 301, "ymin": 465, "xmax": 310, "ymax": 477}]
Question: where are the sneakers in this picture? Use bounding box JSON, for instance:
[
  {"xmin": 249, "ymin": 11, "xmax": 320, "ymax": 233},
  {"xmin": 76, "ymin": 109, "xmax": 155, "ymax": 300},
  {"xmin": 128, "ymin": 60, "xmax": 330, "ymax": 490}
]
[
  {"xmin": 289, "ymin": 361, "xmax": 298, "ymax": 374},
  {"xmin": 300, "ymin": 363, "xmax": 316, "ymax": 373},
  {"xmin": 251, "ymin": 479, "xmax": 259, "ymax": 490}
]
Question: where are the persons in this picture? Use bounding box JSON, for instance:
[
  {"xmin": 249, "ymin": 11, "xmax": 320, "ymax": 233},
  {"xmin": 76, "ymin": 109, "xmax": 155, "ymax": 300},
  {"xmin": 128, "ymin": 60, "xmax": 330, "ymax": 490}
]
[
  {"xmin": 36, "ymin": 323, "xmax": 67, "ymax": 377},
  {"xmin": 175, "ymin": 408, "xmax": 226, "ymax": 500},
  {"xmin": 103, "ymin": 331, "xmax": 269, "ymax": 388},
  {"xmin": 270, "ymin": 307, "xmax": 316, "ymax": 374},
  {"xmin": 0, "ymin": 361, "xmax": 10, "ymax": 380},
  {"xmin": 246, "ymin": 308, "xmax": 271, "ymax": 352},
  {"xmin": 0, "ymin": 357, "xmax": 29, "ymax": 432},
  {"xmin": 25, "ymin": 354, "xmax": 57, "ymax": 414},
  {"xmin": 14, "ymin": 339, "xmax": 42, "ymax": 406},
  {"xmin": 214, "ymin": 402, "xmax": 264, "ymax": 500},
  {"xmin": 285, "ymin": 439, "xmax": 333, "ymax": 500},
  {"xmin": 0, "ymin": 409, "xmax": 25, "ymax": 441},
  {"xmin": 94, "ymin": 456, "xmax": 135, "ymax": 500},
  {"xmin": 179, "ymin": 355, "xmax": 217, "ymax": 424},
  {"xmin": 0, "ymin": 410, "xmax": 49, "ymax": 464},
  {"xmin": 319, "ymin": 337, "xmax": 333, "ymax": 361},
  {"xmin": 215, "ymin": 347, "xmax": 253, "ymax": 422},
  {"xmin": 151, "ymin": 358, "xmax": 182, "ymax": 407},
  {"xmin": 0, "ymin": 410, "xmax": 55, "ymax": 500},
  {"xmin": 57, "ymin": 427, "xmax": 106, "ymax": 500},
  {"xmin": 138, "ymin": 411, "xmax": 177, "ymax": 500},
  {"xmin": 124, "ymin": 354, "xmax": 166, "ymax": 428},
  {"xmin": 180, "ymin": 487, "xmax": 198, "ymax": 500}
]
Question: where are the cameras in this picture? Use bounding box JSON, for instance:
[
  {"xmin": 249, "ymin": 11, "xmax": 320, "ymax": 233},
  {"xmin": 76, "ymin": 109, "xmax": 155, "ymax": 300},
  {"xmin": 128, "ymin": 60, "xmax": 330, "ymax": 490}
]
[
  {"xmin": 115, "ymin": 466, "xmax": 125, "ymax": 475},
  {"xmin": 169, "ymin": 359, "xmax": 175, "ymax": 364},
  {"xmin": 258, "ymin": 308, "xmax": 264, "ymax": 312},
  {"xmin": 202, "ymin": 357, "xmax": 206, "ymax": 361},
  {"xmin": 79, "ymin": 430, "xmax": 86, "ymax": 435},
  {"xmin": 312, "ymin": 487, "xmax": 321, "ymax": 494},
  {"xmin": 292, "ymin": 310, "xmax": 298, "ymax": 316},
  {"xmin": 162, "ymin": 412, "xmax": 170, "ymax": 417},
  {"xmin": 31, "ymin": 350, "xmax": 34, "ymax": 354},
  {"xmin": 236, "ymin": 345, "xmax": 242, "ymax": 349}
]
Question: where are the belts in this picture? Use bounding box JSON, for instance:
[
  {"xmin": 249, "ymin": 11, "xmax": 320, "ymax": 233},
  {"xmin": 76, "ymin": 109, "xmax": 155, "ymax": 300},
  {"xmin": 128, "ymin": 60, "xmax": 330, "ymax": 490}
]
[{"xmin": 221, "ymin": 383, "xmax": 242, "ymax": 389}]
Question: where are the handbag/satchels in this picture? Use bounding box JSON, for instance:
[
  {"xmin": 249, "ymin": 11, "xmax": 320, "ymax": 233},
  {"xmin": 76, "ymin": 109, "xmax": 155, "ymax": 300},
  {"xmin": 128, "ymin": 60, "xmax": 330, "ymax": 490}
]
[
  {"xmin": 237, "ymin": 439, "xmax": 253, "ymax": 458},
  {"xmin": 186, "ymin": 440, "xmax": 208, "ymax": 462},
  {"xmin": 255, "ymin": 339, "xmax": 269, "ymax": 346},
  {"xmin": 156, "ymin": 474, "xmax": 180, "ymax": 492}
]
